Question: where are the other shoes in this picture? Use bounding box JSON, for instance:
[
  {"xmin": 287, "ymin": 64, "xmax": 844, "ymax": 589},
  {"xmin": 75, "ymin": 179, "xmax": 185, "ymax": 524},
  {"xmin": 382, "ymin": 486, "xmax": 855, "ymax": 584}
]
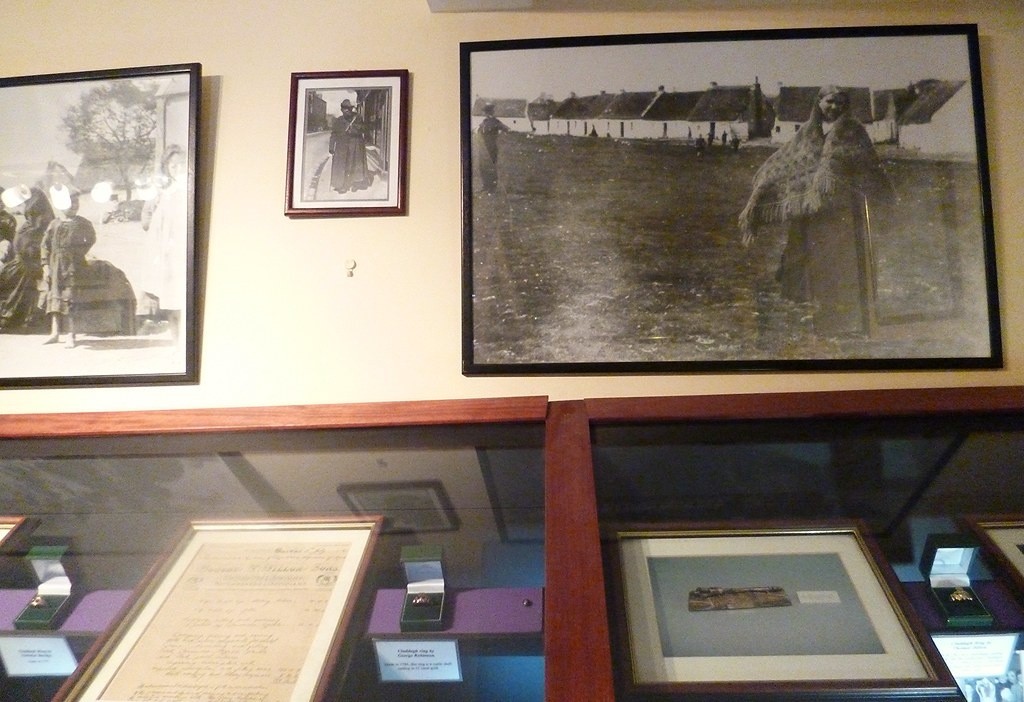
[
  {"xmin": 41, "ymin": 331, "xmax": 60, "ymax": 344},
  {"xmin": 64, "ymin": 333, "xmax": 77, "ymax": 348}
]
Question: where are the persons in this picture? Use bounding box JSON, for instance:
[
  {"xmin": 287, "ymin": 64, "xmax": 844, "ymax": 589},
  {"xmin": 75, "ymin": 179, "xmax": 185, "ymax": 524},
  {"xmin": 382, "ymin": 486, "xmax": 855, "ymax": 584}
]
[
  {"xmin": 330, "ymin": 99, "xmax": 370, "ymax": 194},
  {"xmin": 0, "ymin": 187, "xmax": 96, "ymax": 349},
  {"xmin": 477, "ymin": 104, "xmax": 508, "ymax": 196},
  {"xmin": 740, "ymin": 85, "xmax": 884, "ymax": 304}
]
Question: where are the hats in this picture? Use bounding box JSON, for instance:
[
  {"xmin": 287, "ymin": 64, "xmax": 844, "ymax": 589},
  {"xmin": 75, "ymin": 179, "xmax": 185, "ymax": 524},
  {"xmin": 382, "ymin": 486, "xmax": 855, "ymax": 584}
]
[
  {"xmin": 482, "ymin": 103, "xmax": 495, "ymax": 112},
  {"xmin": 341, "ymin": 99, "xmax": 355, "ymax": 108}
]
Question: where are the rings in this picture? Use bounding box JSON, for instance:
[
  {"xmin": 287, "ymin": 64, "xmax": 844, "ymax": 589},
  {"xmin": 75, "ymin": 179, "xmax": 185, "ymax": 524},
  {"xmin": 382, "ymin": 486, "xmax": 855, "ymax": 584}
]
[
  {"xmin": 951, "ymin": 586, "xmax": 973, "ymax": 601},
  {"xmin": 412, "ymin": 594, "xmax": 433, "ymax": 606},
  {"xmin": 31, "ymin": 598, "xmax": 50, "ymax": 607}
]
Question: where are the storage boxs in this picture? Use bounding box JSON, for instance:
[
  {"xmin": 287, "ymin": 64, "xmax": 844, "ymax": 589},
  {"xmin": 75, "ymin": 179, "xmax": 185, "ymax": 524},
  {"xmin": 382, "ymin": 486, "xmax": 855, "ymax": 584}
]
[
  {"xmin": 397, "ymin": 544, "xmax": 450, "ymax": 632},
  {"xmin": 14, "ymin": 546, "xmax": 84, "ymax": 631},
  {"xmin": 918, "ymin": 531, "xmax": 994, "ymax": 628}
]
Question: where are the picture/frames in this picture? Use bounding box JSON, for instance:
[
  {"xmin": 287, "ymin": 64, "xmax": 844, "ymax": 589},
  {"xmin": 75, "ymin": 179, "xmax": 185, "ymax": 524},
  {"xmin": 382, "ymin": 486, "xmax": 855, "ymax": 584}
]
[
  {"xmin": 50, "ymin": 513, "xmax": 384, "ymax": 702},
  {"xmin": 282, "ymin": 69, "xmax": 411, "ymax": 217},
  {"xmin": 457, "ymin": 24, "xmax": 1002, "ymax": 375},
  {"xmin": 605, "ymin": 516, "xmax": 960, "ymax": 699},
  {"xmin": 0, "ymin": 61, "xmax": 202, "ymax": 386},
  {"xmin": 958, "ymin": 511, "xmax": 1024, "ymax": 618}
]
